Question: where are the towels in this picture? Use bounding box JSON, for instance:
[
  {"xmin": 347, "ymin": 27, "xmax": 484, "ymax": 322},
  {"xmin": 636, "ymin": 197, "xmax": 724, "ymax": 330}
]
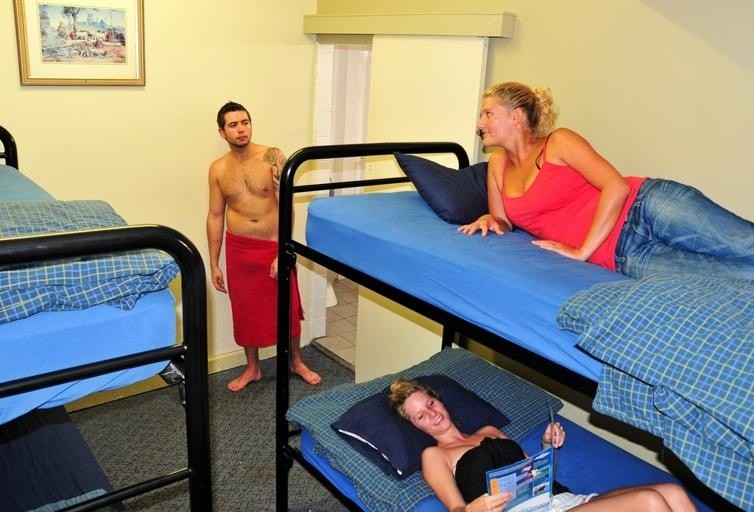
[{"xmin": 225, "ymin": 229, "xmax": 305, "ymax": 347}]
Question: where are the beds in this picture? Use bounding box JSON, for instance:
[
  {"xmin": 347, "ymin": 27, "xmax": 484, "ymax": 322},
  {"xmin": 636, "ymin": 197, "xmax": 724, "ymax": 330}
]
[
  {"xmin": 276, "ymin": 142, "xmax": 754, "ymax": 512},
  {"xmin": 0, "ymin": 125, "xmax": 212, "ymax": 511}
]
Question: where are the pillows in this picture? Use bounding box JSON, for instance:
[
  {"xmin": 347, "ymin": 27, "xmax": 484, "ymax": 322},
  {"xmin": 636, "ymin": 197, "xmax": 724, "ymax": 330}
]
[
  {"xmin": 331, "ymin": 372, "xmax": 509, "ymax": 481},
  {"xmin": 393, "ymin": 150, "xmax": 490, "ymax": 225}
]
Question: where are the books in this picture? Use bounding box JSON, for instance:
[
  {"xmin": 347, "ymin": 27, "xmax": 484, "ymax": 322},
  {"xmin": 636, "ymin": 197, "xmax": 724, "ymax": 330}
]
[{"xmin": 485, "ymin": 395, "xmax": 554, "ymax": 512}]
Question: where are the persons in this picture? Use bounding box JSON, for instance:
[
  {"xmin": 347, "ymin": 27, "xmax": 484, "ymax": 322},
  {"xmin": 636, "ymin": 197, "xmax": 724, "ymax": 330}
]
[
  {"xmin": 458, "ymin": 81, "xmax": 754, "ymax": 280},
  {"xmin": 390, "ymin": 378, "xmax": 697, "ymax": 512},
  {"xmin": 206, "ymin": 101, "xmax": 321, "ymax": 392}
]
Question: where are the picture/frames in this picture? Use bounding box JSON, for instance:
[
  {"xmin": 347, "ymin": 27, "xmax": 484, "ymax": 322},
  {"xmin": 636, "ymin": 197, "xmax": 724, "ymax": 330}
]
[{"xmin": 13, "ymin": 0, "xmax": 145, "ymax": 86}]
